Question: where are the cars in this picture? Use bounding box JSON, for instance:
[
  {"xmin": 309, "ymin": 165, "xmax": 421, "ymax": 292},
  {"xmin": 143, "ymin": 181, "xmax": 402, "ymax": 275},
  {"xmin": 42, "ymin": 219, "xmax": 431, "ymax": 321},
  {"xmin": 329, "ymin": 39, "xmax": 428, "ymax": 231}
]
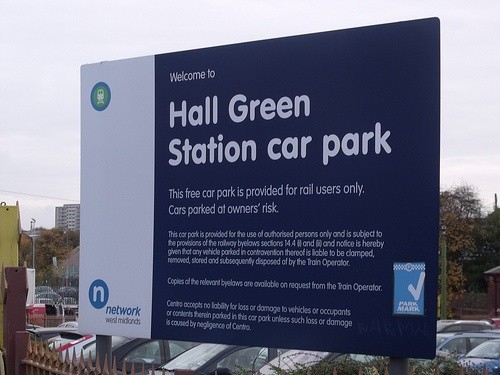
[
  {"xmin": 436, "ymin": 317, "xmax": 500, "ymax": 375},
  {"xmin": 35, "ymin": 286, "xmax": 79, "ymax": 316},
  {"xmin": 25, "ymin": 322, "xmax": 436, "ymax": 375}
]
